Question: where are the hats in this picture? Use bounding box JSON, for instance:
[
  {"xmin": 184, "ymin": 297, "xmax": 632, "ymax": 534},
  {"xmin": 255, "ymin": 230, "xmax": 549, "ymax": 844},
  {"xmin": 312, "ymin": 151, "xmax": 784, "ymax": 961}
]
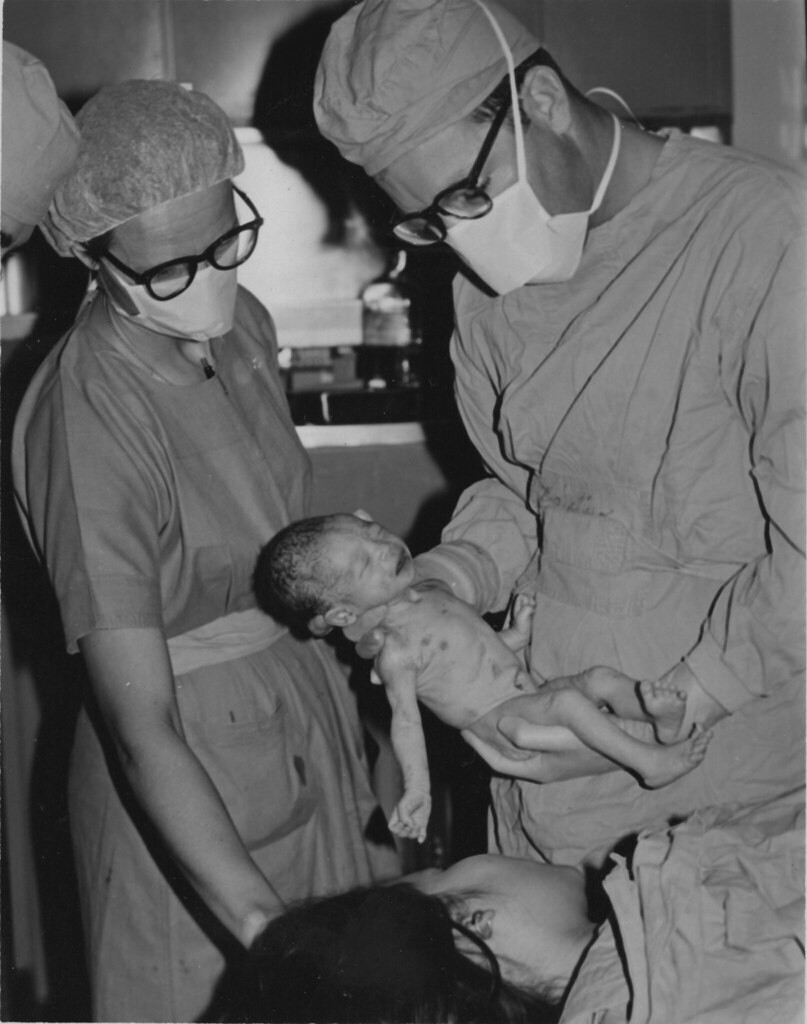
[
  {"xmin": 2, "ymin": 40, "xmax": 81, "ymax": 226},
  {"xmin": 312, "ymin": 1, "xmax": 542, "ymax": 175}
]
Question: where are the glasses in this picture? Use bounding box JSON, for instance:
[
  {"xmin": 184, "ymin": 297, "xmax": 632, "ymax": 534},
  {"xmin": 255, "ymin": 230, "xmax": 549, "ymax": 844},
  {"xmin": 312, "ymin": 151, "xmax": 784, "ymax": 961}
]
[
  {"xmin": 87, "ymin": 180, "xmax": 264, "ymax": 300},
  {"xmin": 391, "ymin": 84, "xmax": 519, "ymax": 250}
]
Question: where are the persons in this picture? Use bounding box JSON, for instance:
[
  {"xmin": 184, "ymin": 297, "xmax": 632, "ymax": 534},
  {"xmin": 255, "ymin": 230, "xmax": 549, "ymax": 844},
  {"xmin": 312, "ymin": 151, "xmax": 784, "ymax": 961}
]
[{"xmin": 0, "ymin": 0, "xmax": 807, "ymax": 1024}]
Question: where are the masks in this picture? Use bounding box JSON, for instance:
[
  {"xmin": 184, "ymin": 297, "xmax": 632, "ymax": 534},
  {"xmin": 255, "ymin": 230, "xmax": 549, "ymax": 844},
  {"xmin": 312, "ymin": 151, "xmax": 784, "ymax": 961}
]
[
  {"xmin": 97, "ymin": 236, "xmax": 240, "ymax": 341},
  {"xmin": 443, "ymin": 125, "xmax": 622, "ymax": 296}
]
